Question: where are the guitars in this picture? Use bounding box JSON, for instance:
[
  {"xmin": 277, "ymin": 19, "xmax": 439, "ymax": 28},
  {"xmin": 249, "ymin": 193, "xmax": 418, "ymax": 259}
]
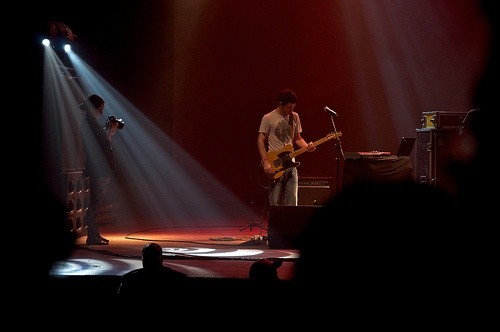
[{"xmin": 260, "ymin": 131, "xmax": 343, "ymax": 179}]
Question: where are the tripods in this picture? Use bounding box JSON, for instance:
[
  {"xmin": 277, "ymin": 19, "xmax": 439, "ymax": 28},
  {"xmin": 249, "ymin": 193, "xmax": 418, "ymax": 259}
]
[{"xmin": 238, "ymin": 177, "xmax": 268, "ymax": 232}]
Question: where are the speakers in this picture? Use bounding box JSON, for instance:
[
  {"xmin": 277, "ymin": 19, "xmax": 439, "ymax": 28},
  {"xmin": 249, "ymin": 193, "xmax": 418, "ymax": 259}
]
[
  {"xmin": 297, "ymin": 176, "xmax": 336, "ymax": 206},
  {"xmin": 267, "ymin": 204, "xmax": 319, "ymax": 251}
]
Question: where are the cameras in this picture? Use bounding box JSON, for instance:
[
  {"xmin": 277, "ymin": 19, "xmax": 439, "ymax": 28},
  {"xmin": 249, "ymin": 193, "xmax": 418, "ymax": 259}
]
[{"xmin": 106, "ymin": 116, "xmax": 125, "ymax": 129}]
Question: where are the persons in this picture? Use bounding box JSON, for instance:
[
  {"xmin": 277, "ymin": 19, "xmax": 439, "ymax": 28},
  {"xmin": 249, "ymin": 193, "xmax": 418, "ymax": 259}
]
[
  {"xmin": 249, "ymin": 259, "xmax": 280, "ymax": 281},
  {"xmin": 78, "ymin": 95, "xmax": 118, "ymax": 244},
  {"xmin": 257, "ymin": 90, "xmax": 315, "ymax": 205},
  {"xmin": 470, "ymin": 0, "xmax": 500, "ymax": 185},
  {"xmin": 283, "ymin": 174, "xmax": 500, "ymax": 332},
  {"xmin": 117, "ymin": 243, "xmax": 188, "ymax": 295}
]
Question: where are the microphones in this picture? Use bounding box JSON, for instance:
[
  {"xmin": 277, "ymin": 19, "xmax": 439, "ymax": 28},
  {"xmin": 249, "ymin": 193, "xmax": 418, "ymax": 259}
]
[{"xmin": 325, "ymin": 106, "xmax": 339, "ymax": 117}]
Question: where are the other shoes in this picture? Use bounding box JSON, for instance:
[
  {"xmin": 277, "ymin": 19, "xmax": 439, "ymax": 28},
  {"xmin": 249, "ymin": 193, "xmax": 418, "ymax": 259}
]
[{"xmin": 86, "ymin": 234, "xmax": 109, "ymax": 245}]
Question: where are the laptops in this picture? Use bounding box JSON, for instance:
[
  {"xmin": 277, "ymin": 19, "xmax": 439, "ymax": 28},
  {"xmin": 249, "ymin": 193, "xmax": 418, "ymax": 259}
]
[{"xmin": 392, "ymin": 136, "xmax": 416, "ymax": 157}]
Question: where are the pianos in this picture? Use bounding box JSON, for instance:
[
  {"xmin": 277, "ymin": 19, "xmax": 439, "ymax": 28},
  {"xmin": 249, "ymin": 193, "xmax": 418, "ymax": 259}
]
[{"xmin": 344, "ymin": 150, "xmax": 410, "ymax": 180}]
